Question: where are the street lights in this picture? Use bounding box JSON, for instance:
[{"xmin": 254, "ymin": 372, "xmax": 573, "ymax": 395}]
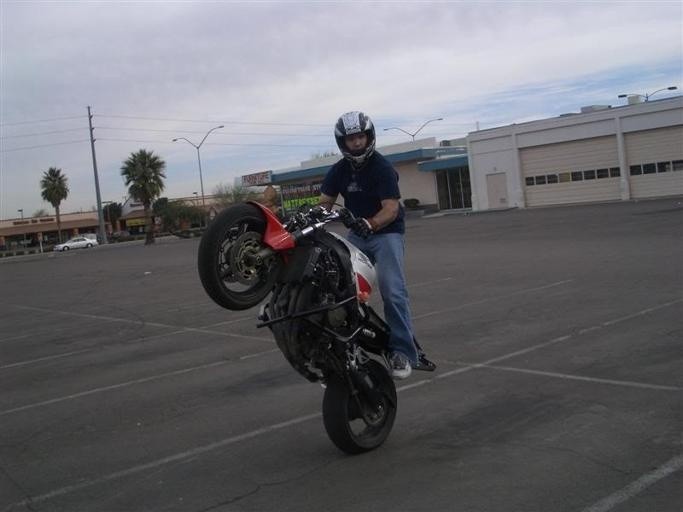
[
  {"xmin": 384, "ymin": 118, "xmax": 443, "ymax": 148},
  {"xmin": 618, "ymin": 87, "xmax": 677, "ymax": 102},
  {"xmin": 173, "ymin": 125, "xmax": 225, "ymax": 229}
]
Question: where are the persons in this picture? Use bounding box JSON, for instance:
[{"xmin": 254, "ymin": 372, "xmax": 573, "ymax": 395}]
[{"xmin": 312, "ymin": 110, "xmax": 424, "ymax": 380}]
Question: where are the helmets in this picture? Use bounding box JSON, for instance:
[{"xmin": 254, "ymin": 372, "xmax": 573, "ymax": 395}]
[{"xmin": 334, "ymin": 111, "xmax": 377, "ymax": 165}]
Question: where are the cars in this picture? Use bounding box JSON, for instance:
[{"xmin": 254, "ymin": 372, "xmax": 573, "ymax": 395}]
[{"xmin": 54, "ymin": 237, "xmax": 98, "ymax": 252}]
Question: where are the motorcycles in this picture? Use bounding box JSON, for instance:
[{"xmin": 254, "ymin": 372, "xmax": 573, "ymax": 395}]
[{"xmin": 198, "ymin": 199, "xmax": 437, "ymax": 455}]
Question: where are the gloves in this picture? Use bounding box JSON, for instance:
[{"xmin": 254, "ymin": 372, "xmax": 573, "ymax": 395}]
[{"xmin": 351, "ymin": 218, "xmax": 376, "ymax": 240}]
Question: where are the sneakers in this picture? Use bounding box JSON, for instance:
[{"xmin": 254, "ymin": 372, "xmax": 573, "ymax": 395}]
[{"xmin": 389, "ymin": 354, "xmax": 412, "ymax": 380}]
